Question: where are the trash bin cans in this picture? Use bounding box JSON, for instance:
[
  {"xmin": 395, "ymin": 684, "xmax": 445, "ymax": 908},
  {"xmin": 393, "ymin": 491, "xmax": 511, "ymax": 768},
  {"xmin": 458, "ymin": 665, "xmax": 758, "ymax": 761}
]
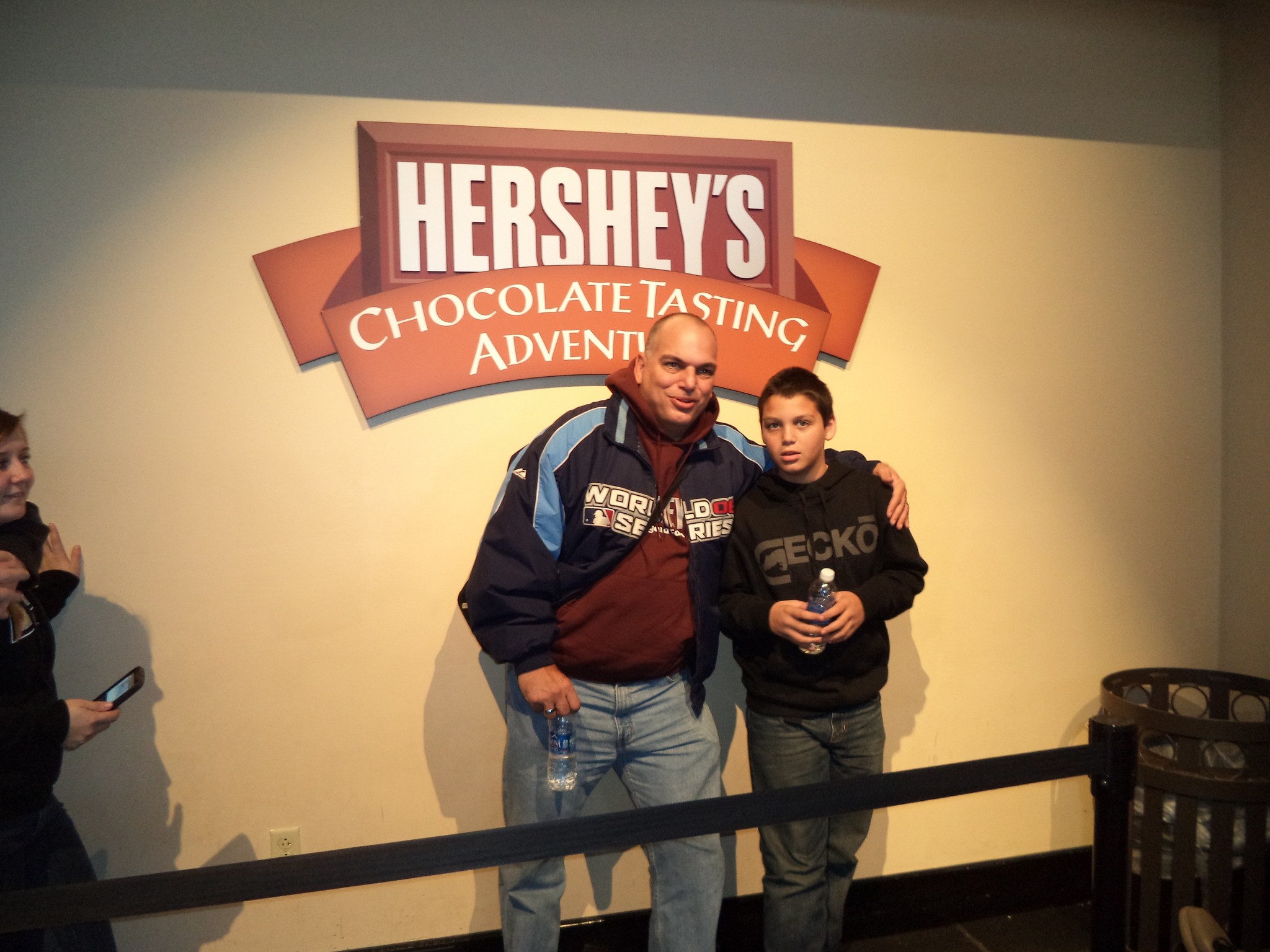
[{"xmin": 1101, "ymin": 665, "xmax": 1268, "ymax": 951}]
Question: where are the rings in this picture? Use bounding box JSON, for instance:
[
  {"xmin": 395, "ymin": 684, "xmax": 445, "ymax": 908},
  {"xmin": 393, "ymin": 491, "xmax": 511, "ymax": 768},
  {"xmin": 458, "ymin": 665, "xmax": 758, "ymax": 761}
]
[{"xmin": 542, "ymin": 707, "xmax": 555, "ymax": 714}]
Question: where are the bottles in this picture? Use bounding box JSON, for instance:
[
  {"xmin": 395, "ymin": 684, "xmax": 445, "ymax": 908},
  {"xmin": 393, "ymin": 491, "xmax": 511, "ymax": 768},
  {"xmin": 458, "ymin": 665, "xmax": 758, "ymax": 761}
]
[
  {"xmin": 799, "ymin": 568, "xmax": 837, "ymax": 655},
  {"xmin": 547, "ymin": 711, "xmax": 578, "ymax": 791}
]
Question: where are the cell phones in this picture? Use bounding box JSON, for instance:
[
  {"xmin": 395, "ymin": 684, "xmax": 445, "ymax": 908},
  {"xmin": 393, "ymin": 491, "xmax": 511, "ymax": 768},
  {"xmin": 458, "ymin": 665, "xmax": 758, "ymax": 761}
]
[{"xmin": 93, "ymin": 666, "xmax": 146, "ymax": 710}]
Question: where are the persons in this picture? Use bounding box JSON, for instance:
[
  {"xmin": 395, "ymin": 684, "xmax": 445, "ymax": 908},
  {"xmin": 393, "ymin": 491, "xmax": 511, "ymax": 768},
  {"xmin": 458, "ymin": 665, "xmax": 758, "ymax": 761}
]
[
  {"xmin": 715, "ymin": 365, "xmax": 930, "ymax": 952},
  {"xmin": 0, "ymin": 408, "xmax": 120, "ymax": 952},
  {"xmin": 457, "ymin": 311, "xmax": 911, "ymax": 952}
]
